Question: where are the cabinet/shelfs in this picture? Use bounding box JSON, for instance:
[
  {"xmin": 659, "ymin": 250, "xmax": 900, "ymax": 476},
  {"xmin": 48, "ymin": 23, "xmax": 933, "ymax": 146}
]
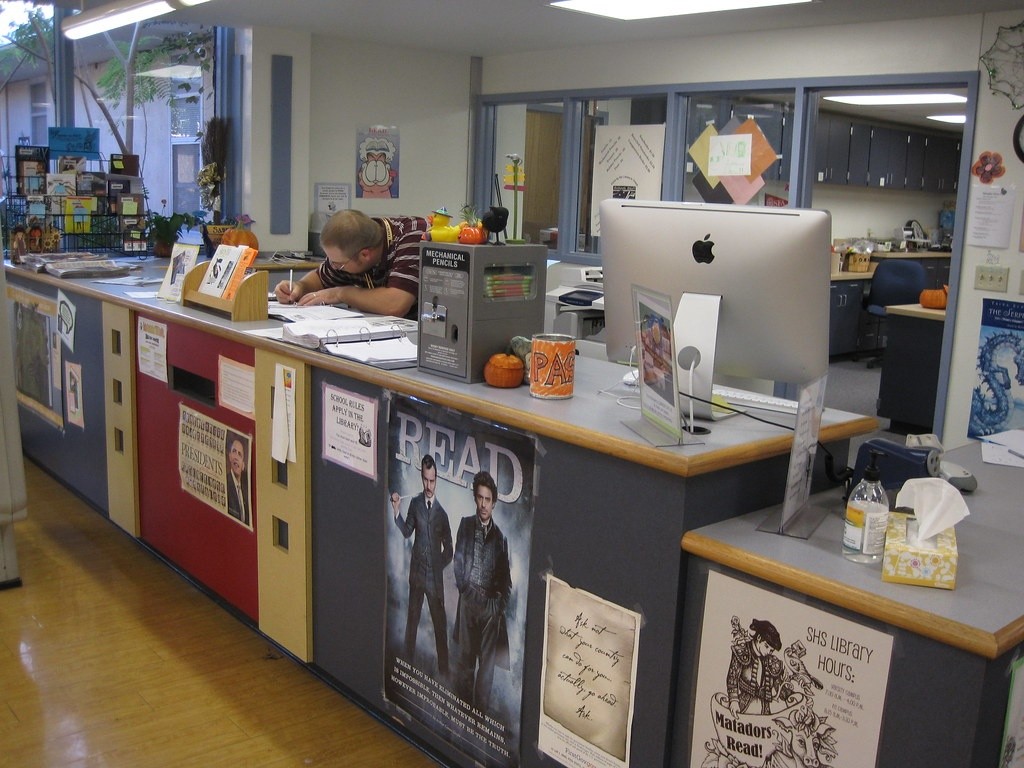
[
  {"xmin": 829, "ymin": 279, "xmax": 888, "ymax": 362},
  {"xmin": 732, "ymin": 101, "xmax": 962, "ymax": 194}
]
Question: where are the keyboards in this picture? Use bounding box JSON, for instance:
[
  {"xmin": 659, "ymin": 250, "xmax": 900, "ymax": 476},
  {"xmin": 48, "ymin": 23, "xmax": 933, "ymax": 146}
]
[{"xmin": 622, "ymin": 367, "xmax": 823, "ymax": 417}]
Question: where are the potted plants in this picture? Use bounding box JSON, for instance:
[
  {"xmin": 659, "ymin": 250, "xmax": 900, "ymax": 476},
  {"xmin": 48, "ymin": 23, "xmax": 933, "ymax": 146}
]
[{"xmin": 150, "ymin": 212, "xmax": 185, "ymax": 258}]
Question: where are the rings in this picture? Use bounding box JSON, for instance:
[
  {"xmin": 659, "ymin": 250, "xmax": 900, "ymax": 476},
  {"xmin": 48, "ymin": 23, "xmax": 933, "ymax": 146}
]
[{"xmin": 313, "ymin": 294, "xmax": 316, "ymax": 297}]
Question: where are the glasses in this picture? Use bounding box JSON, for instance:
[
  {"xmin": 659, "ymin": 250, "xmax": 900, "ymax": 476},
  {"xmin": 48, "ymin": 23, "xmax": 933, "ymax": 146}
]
[{"xmin": 335, "ymin": 246, "xmax": 369, "ymax": 271}]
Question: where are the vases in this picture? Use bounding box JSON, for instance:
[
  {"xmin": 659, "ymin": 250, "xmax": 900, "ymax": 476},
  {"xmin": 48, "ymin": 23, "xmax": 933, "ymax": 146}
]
[{"xmin": 200, "ymin": 225, "xmax": 235, "ymax": 254}]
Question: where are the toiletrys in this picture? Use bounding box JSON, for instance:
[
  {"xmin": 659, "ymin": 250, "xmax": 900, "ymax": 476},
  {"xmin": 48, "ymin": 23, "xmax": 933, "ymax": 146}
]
[{"xmin": 841, "ymin": 450, "xmax": 891, "ymax": 566}]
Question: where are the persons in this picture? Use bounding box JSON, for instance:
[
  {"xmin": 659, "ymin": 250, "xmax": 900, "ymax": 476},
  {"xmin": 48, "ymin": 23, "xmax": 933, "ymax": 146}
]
[
  {"xmin": 274, "ymin": 209, "xmax": 427, "ymax": 320},
  {"xmin": 451, "ymin": 471, "xmax": 511, "ymax": 709},
  {"xmin": 227, "ymin": 438, "xmax": 249, "ymax": 526},
  {"xmin": 390, "ymin": 454, "xmax": 454, "ymax": 679}
]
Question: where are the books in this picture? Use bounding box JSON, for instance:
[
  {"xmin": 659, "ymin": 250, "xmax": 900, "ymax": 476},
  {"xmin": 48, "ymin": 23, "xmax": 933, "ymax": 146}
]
[
  {"xmin": 14, "ymin": 143, "xmax": 147, "ymax": 278},
  {"xmin": 281, "ymin": 319, "xmax": 418, "ymax": 371}
]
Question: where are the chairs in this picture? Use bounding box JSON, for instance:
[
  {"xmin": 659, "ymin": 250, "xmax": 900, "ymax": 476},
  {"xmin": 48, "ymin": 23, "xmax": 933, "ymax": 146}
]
[{"xmin": 862, "ymin": 258, "xmax": 927, "ymax": 369}]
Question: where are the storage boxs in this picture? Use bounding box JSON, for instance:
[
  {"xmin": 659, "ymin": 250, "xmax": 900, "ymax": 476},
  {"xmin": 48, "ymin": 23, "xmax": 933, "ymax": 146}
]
[{"xmin": 64, "ymin": 196, "xmax": 92, "ymax": 233}]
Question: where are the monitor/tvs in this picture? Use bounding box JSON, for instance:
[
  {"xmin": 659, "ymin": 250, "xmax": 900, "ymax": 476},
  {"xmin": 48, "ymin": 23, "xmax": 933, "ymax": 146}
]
[{"xmin": 601, "ymin": 194, "xmax": 832, "ymax": 420}]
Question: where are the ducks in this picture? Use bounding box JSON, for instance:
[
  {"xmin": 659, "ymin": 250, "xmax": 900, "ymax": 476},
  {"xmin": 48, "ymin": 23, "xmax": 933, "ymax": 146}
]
[{"xmin": 425, "ymin": 212, "xmax": 461, "ymax": 243}]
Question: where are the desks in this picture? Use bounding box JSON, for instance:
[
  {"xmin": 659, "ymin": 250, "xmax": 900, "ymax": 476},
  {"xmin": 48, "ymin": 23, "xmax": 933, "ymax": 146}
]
[
  {"xmin": 871, "ymin": 250, "xmax": 951, "ymax": 291},
  {"xmin": 875, "ymin": 302, "xmax": 946, "ymax": 435},
  {"xmin": 671, "ymin": 427, "xmax": 1024, "ymax": 768}
]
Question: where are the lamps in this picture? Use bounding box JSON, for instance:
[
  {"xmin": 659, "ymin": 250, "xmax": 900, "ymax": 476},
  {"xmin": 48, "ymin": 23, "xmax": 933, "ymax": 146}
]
[{"xmin": 59, "ymin": 0, "xmax": 212, "ymax": 40}]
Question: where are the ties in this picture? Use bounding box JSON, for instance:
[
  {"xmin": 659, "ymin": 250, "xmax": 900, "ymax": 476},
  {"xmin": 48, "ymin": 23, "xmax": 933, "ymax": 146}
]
[
  {"xmin": 236, "ymin": 485, "xmax": 244, "ymax": 522},
  {"xmin": 427, "ymin": 500, "xmax": 431, "ymax": 510}
]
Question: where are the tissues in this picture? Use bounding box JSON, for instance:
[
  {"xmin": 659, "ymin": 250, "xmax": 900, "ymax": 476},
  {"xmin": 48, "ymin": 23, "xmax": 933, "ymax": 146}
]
[{"xmin": 881, "ymin": 477, "xmax": 972, "ymax": 590}]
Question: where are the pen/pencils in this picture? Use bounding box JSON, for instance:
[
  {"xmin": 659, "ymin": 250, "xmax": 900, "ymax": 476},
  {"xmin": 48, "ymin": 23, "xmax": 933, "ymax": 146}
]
[{"xmin": 290, "ymin": 269, "xmax": 293, "ymax": 292}]
